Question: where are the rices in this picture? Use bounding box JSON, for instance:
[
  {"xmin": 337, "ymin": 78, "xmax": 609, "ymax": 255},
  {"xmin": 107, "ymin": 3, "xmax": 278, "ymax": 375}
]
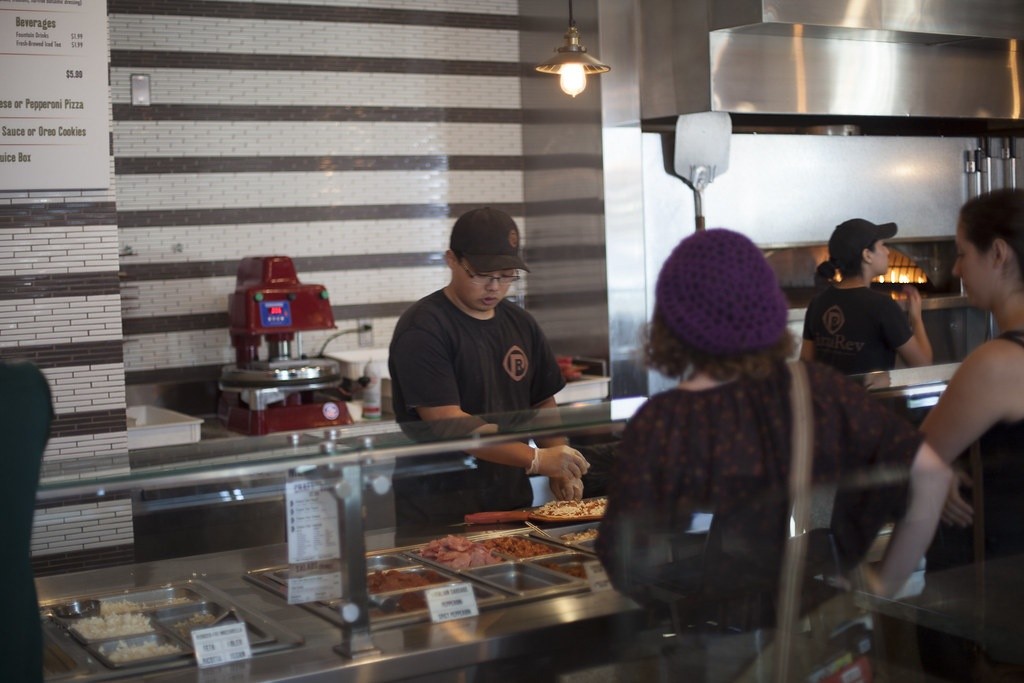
[
  {"xmin": 67, "ymin": 612, "xmax": 154, "ymax": 640},
  {"xmin": 100, "ymin": 599, "xmax": 146, "ymax": 614},
  {"xmin": 98, "ymin": 639, "xmax": 182, "ymax": 662}
]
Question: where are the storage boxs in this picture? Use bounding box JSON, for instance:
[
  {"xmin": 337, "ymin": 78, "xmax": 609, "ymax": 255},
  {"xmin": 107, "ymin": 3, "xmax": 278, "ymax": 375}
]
[
  {"xmin": 124, "ymin": 404, "xmax": 206, "ymax": 448},
  {"xmin": 553, "ymin": 374, "xmax": 613, "ymax": 406},
  {"xmin": 322, "ymin": 348, "xmax": 384, "ymax": 381}
]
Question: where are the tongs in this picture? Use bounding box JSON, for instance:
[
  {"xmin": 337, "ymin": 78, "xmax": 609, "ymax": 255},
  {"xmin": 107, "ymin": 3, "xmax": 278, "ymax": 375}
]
[{"xmin": 209, "ymin": 603, "xmax": 244, "ymax": 626}]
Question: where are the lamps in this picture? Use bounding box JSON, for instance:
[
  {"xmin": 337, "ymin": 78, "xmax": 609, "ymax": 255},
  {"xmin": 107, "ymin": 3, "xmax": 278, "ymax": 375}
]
[{"xmin": 532, "ymin": 0, "xmax": 612, "ymax": 98}]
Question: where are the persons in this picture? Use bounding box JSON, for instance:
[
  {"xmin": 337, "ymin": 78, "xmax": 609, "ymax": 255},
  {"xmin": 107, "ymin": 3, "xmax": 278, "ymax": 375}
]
[
  {"xmin": 596, "ymin": 187, "xmax": 1024, "ymax": 683},
  {"xmin": 388, "ymin": 208, "xmax": 590, "ymax": 548}
]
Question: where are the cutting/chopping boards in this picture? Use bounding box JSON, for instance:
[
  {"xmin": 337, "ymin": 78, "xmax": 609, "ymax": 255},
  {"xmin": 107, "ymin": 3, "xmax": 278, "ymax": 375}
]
[{"xmin": 518, "ymin": 494, "xmax": 604, "ymax": 521}]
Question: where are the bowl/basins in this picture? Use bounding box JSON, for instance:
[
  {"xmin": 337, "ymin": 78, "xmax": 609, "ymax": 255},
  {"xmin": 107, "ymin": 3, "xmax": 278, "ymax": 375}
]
[{"xmin": 53, "ymin": 599, "xmax": 101, "ymax": 619}]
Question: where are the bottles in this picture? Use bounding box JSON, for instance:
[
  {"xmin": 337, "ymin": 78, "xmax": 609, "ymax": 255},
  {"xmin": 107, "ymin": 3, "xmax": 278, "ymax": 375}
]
[{"xmin": 361, "ymin": 354, "xmax": 382, "ymax": 421}]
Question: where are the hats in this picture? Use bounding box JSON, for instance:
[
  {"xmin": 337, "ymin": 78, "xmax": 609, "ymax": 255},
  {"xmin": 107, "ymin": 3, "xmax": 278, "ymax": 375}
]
[
  {"xmin": 450, "ymin": 206, "xmax": 531, "ymax": 273},
  {"xmin": 828, "ymin": 218, "xmax": 897, "ymax": 259},
  {"xmin": 656, "ymin": 228, "xmax": 787, "ymax": 356}
]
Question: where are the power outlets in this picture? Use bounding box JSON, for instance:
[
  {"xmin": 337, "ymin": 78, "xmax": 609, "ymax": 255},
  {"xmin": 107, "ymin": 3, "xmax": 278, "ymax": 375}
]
[{"xmin": 357, "ymin": 317, "xmax": 373, "ymax": 348}]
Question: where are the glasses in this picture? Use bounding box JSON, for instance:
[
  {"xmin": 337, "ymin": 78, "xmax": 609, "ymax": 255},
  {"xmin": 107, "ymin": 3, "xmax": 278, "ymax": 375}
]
[{"xmin": 458, "ymin": 256, "xmax": 521, "ymax": 285}]
